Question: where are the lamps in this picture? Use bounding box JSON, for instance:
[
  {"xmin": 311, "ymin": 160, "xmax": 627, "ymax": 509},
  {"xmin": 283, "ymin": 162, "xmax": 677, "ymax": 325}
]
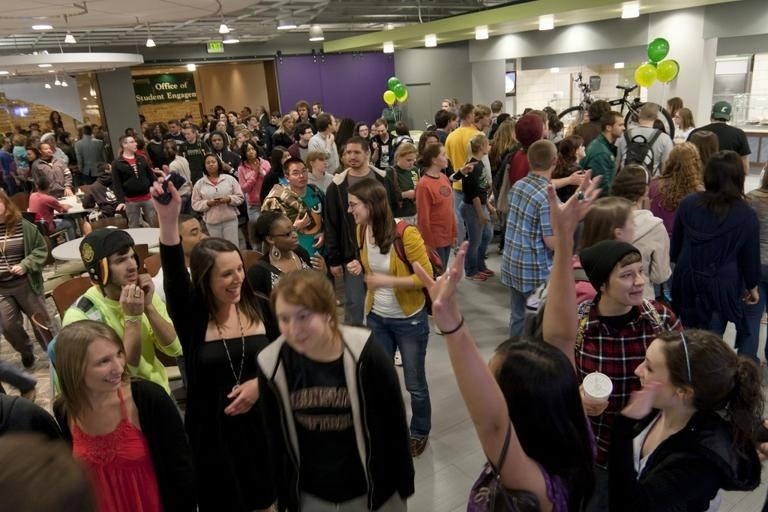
[
  {"xmin": 146, "ymin": 17, "xmax": 156, "ymax": 48},
  {"xmin": 30, "ymin": 24, "xmax": 77, "ymax": 89},
  {"xmin": 218, "ymin": 7, "xmax": 240, "ymax": 44},
  {"xmin": 382, "ymin": 1, "xmax": 641, "ymax": 56},
  {"xmin": 276, "ymin": 9, "xmax": 324, "ymax": 42},
  {"xmin": 187, "ymin": 63, "xmax": 197, "ymax": 72}
]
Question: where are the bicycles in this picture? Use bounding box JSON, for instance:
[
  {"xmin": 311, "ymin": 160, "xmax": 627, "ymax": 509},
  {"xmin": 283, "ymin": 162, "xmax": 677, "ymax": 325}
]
[{"xmin": 555, "ymin": 71, "xmax": 675, "ymax": 143}]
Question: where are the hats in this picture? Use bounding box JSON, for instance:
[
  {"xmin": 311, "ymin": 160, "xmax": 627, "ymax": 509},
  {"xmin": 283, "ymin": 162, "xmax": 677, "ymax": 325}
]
[
  {"xmin": 711, "ymin": 100, "xmax": 731, "ymax": 120},
  {"xmin": 78, "ymin": 229, "xmax": 139, "ymax": 287},
  {"xmin": 514, "ymin": 114, "xmax": 546, "ymax": 148},
  {"xmin": 578, "ymin": 241, "xmax": 642, "ymax": 291}
]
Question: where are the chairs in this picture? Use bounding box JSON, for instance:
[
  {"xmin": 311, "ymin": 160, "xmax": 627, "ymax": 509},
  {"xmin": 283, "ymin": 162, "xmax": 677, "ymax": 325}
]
[
  {"xmin": 20, "ymin": 209, "xmax": 90, "ymax": 248},
  {"xmin": 32, "ymin": 277, "xmax": 93, "ymax": 345},
  {"xmin": 134, "ymin": 244, "xmax": 161, "ymax": 278},
  {"xmin": 240, "ymin": 241, "xmax": 265, "ymax": 274}
]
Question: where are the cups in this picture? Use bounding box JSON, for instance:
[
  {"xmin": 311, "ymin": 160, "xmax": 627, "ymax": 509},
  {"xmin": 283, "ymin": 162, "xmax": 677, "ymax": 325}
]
[{"xmin": 583, "ymin": 373, "xmax": 613, "ymax": 401}]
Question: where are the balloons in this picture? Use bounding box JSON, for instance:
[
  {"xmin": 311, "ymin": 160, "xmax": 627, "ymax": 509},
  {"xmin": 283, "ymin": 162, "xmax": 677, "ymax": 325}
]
[
  {"xmin": 383, "ymin": 76, "xmax": 408, "ymax": 106},
  {"xmin": 635, "ymin": 37, "xmax": 679, "ymax": 90}
]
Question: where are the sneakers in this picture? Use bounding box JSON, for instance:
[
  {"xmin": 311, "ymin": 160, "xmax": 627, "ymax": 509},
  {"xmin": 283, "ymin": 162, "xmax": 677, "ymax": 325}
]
[
  {"xmin": 18, "ymin": 342, "xmax": 35, "ymax": 369},
  {"xmin": 409, "ymin": 434, "xmax": 429, "ymax": 455},
  {"xmin": 466, "ymin": 270, "xmax": 495, "ymax": 283}
]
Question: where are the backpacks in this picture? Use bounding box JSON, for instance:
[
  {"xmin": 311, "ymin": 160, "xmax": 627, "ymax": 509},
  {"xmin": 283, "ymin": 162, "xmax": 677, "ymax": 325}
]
[
  {"xmin": 415, "ymin": 248, "xmax": 443, "ymax": 315},
  {"xmin": 621, "ymin": 126, "xmax": 662, "ymax": 176}
]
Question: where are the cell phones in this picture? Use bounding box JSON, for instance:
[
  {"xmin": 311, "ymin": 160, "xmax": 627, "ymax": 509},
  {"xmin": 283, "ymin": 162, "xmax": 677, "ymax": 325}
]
[{"xmin": 155, "ymin": 171, "xmax": 187, "ymax": 205}]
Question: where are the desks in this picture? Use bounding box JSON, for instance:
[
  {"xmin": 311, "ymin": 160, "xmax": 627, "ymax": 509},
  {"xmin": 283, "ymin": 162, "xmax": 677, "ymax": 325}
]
[
  {"xmin": 52, "ymin": 227, "xmax": 162, "ymax": 278},
  {"xmin": 741, "ymin": 125, "xmax": 768, "ymax": 167}
]
